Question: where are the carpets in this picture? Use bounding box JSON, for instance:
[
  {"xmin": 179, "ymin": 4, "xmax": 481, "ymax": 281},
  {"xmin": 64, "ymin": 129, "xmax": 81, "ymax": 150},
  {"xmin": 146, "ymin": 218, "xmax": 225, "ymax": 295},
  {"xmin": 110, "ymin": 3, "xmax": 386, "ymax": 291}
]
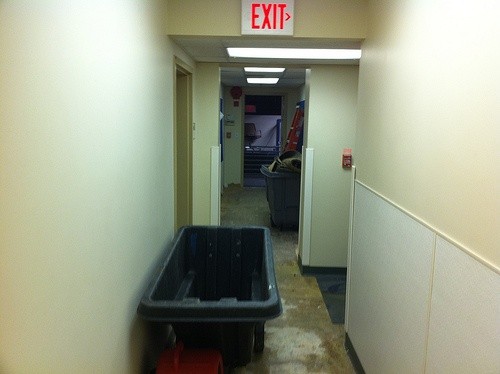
[{"xmin": 315, "ymin": 273, "xmax": 346, "ymax": 324}]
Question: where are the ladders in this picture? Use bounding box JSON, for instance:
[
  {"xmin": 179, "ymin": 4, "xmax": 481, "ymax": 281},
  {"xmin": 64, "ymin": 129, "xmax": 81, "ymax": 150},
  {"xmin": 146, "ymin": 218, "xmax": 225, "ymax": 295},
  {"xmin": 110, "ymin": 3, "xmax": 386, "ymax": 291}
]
[{"xmin": 283, "ymin": 107, "xmax": 303, "ymax": 154}]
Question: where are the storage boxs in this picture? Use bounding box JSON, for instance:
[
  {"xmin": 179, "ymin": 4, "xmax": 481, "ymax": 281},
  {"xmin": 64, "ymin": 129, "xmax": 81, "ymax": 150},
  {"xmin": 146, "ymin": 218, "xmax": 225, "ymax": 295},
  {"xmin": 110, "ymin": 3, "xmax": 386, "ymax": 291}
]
[
  {"xmin": 138, "ymin": 224, "xmax": 283, "ymax": 367},
  {"xmin": 259, "ymin": 164, "xmax": 300, "ymax": 230}
]
